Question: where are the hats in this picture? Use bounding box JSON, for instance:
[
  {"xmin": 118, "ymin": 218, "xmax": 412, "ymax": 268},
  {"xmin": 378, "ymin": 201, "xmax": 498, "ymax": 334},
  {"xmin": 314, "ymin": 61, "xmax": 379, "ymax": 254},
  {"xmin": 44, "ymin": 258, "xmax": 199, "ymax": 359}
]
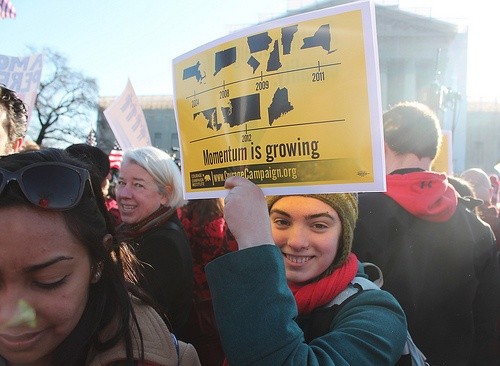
[
  {"xmin": 266, "ymin": 192, "xmax": 358, "ymax": 284},
  {"xmin": 448, "ymin": 177, "xmax": 484, "ymax": 207},
  {"xmin": 462, "ymin": 168, "xmax": 491, "ymax": 203}
]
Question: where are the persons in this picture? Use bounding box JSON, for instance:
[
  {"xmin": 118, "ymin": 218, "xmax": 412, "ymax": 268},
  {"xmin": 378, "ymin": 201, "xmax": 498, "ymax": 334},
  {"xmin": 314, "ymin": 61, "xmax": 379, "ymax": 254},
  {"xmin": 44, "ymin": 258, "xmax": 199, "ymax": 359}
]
[
  {"xmin": 109, "ymin": 146, "xmax": 194, "ymax": 349},
  {"xmin": 0, "ymin": 84, "xmax": 40, "ymax": 157},
  {"xmin": 63, "ymin": 143, "xmax": 123, "ymax": 226},
  {"xmin": 0, "ymin": 147, "xmax": 201, "ymax": 366},
  {"xmin": 488, "ymin": 174, "xmax": 499, "ymax": 207},
  {"xmin": 459, "ymin": 168, "xmax": 499, "ymax": 250},
  {"xmin": 205, "ymin": 175, "xmax": 408, "ymax": 366},
  {"xmin": 352, "ymin": 101, "xmax": 499, "ymax": 366},
  {"xmin": 174, "ymin": 198, "xmax": 239, "ymax": 366}
]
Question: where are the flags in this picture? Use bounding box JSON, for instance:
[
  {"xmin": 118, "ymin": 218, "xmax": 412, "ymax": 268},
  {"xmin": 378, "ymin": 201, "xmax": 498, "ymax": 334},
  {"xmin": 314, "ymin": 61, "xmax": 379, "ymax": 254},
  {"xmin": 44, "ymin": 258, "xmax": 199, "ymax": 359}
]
[
  {"xmin": 107, "ymin": 142, "xmax": 122, "ymax": 171},
  {"xmin": 1, "ymin": 0, "xmax": 18, "ymax": 19}
]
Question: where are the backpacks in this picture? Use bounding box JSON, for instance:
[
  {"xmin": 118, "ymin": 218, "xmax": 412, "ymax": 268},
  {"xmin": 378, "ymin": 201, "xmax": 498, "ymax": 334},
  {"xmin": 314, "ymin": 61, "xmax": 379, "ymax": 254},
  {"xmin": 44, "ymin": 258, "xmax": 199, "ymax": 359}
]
[{"xmin": 322, "ymin": 262, "xmax": 430, "ymax": 366}]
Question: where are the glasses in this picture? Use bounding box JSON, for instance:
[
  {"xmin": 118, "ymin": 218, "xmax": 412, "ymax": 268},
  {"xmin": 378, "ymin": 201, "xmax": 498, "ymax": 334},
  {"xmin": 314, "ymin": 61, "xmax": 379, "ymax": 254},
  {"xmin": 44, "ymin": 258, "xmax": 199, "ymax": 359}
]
[
  {"xmin": 0, "ymin": 85, "xmax": 27, "ymax": 117},
  {"xmin": 0, "ymin": 161, "xmax": 96, "ymax": 212}
]
